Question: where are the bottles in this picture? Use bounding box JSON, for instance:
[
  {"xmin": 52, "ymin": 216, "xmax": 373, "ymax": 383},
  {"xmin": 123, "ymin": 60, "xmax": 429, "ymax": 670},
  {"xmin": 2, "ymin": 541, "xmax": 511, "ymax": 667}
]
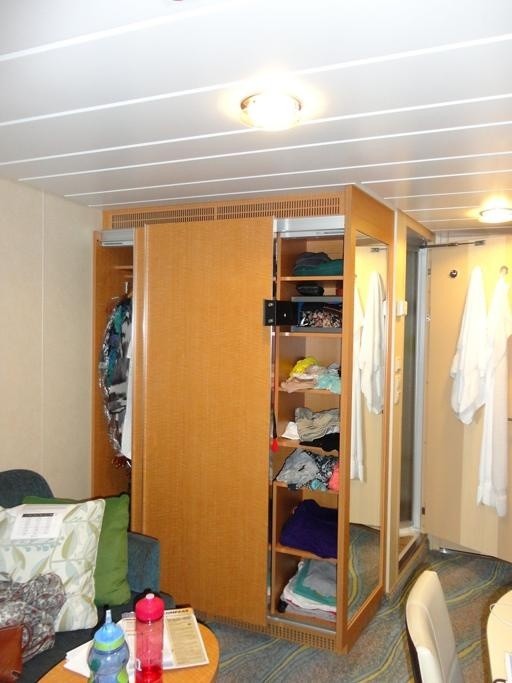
[
  {"xmin": 135, "ymin": 592, "xmax": 165, "ymax": 683},
  {"xmin": 87, "ymin": 608, "xmax": 130, "ymax": 683}
]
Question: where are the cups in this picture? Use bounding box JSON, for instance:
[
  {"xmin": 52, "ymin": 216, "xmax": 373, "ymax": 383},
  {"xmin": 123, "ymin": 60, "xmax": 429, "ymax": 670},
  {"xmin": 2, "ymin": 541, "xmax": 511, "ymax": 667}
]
[{"xmin": 0, "ymin": 623, "xmax": 33, "ymax": 683}]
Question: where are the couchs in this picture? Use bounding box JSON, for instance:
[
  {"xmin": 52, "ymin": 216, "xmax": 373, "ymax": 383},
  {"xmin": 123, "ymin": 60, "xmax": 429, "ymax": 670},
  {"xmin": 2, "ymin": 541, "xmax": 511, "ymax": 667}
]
[{"xmin": 1, "ymin": 468, "xmax": 160, "ymax": 682}]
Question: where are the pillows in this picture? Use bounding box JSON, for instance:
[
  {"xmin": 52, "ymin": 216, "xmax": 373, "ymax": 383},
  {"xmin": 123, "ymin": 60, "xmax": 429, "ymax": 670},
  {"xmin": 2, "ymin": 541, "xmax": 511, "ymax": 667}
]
[{"xmin": 2, "ymin": 494, "xmax": 132, "ymax": 681}]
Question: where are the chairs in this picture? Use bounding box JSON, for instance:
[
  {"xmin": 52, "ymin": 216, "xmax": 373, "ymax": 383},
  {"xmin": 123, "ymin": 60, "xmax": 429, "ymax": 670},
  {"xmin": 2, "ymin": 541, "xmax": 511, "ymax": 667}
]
[{"xmin": 396, "ymin": 563, "xmax": 509, "ymax": 683}]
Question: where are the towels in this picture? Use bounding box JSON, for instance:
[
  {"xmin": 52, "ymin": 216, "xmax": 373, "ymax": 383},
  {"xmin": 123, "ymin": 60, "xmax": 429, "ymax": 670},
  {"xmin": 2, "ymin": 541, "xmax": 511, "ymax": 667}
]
[{"xmin": 446, "ymin": 263, "xmax": 510, "ymax": 519}]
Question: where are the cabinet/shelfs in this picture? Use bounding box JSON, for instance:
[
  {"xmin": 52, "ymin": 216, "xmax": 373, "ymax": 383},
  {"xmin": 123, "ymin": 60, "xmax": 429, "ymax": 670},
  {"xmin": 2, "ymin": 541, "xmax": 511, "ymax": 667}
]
[{"xmin": 91, "ymin": 215, "xmax": 391, "ymax": 655}]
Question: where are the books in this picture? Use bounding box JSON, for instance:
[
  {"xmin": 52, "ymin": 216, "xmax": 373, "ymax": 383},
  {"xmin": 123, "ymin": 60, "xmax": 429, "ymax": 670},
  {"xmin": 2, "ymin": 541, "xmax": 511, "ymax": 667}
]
[{"xmin": 65, "ymin": 604, "xmax": 210, "ymax": 683}]
